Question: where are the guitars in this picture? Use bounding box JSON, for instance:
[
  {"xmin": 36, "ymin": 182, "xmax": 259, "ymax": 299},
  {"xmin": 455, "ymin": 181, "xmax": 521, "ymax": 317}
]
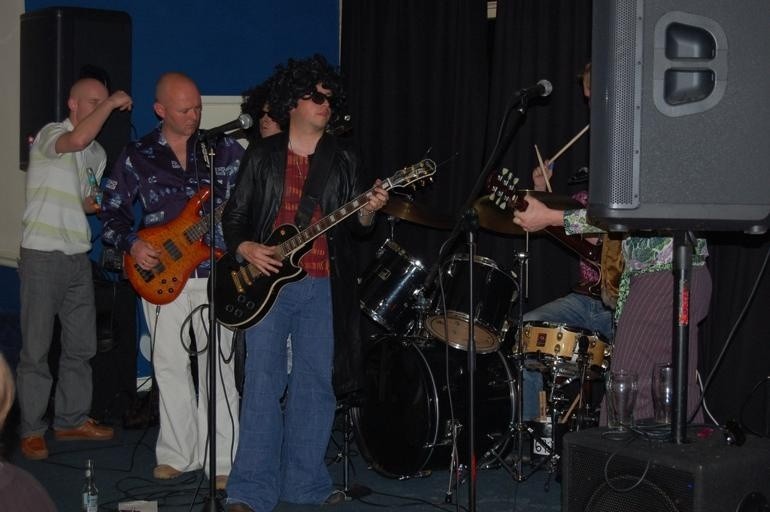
[
  {"xmin": 126, "ymin": 187, "xmax": 233, "ymax": 304},
  {"xmin": 207, "ymin": 158, "xmax": 437, "ymax": 330},
  {"xmin": 481, "ymin": 166, "xmax": 624, "ymax": 308}
]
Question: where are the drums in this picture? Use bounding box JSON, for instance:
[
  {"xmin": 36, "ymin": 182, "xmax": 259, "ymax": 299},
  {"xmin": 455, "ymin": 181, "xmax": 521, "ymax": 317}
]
[
  {"xmin": 519, "ymin": 321, "xmax": 609, "ymax": 380},
  {"xmin": 427, "ymin": 253, "xmax": 520, "ymax": 355},
  {"xmin": 356, "ymin": 238, "xmax": 436, "ymax": 338},
  {"xmin": 350, "ymin": 335, "xmax": 517, "ymax": 478}
]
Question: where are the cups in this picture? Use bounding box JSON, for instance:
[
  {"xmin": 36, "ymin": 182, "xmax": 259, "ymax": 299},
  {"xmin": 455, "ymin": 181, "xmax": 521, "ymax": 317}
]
[
  {"xmin": 652, "ymin": 364, "xmax": 672, "ymax": 427},
  {"xmin": 601, "ymin": 369, "xmax": 639, "ymax": 433}
]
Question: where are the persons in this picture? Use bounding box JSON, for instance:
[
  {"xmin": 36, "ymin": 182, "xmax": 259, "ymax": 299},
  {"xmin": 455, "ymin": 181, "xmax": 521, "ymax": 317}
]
[
  {"xmin": 255, "ymin": 89, "xmax": 281, "ymax": 137},
  {"xmin": 18, "ymin": 77, "xmax": 132, "ymax": 460},
  {"xmin": 224, "ymin": 57, "xmax": 389, "ymax": 509},
  {"xmin": 103, "ymin": 73, "xmax": 242, "ymax": 491},
  {"xmin": 511, "ymin": 195, "xmax": 713, "ymax": 432},
  {"xmin": 497, "ymin": 158, "xmax": 603, "ymax": 465}
]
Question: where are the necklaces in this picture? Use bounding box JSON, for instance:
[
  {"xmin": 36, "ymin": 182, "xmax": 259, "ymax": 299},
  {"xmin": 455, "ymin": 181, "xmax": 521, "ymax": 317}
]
[{"xmin": 289, "ymin": 140, "xmax": 306, "ymax": 183}]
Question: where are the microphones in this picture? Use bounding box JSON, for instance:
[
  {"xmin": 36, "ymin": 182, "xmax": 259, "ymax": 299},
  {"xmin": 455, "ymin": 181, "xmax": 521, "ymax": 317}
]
[{"xmin": 196, "ymin": 114, "xmax": 253, "ymax": 142}]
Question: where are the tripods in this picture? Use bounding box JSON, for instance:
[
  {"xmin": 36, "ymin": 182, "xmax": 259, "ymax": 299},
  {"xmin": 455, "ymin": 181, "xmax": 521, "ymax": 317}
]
[{"xmin": 450, "ymin": 249, "xmax": 558, "ymax": 492}]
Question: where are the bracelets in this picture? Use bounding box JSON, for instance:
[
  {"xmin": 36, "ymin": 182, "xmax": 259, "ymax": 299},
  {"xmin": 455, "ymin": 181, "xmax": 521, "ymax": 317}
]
[{"xmin": 358, "ymin": 207, "xmax": 375, "ymax": 217}]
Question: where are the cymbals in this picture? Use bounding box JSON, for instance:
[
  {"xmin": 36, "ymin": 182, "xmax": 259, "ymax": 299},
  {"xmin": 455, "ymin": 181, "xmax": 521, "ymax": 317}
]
[
  {"xmin": 385, "ymin": 192, "xmax": 451, "ymax": 230},
  {"xmin": 474, "ymin": 191, "xmax": 587, "ymax": 235}
]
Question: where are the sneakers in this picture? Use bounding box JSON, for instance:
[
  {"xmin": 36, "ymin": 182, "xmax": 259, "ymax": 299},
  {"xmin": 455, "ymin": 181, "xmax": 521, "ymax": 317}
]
[
  {"xmin": 210, "ymin": 474, "xmax": 229, "ymax": 490},
  {"xmin": 224, "ymin": 488, "xmax": 346, "ymax": 511},
  {"xmin": 152, "ymin": 464, "xmax": 185, "ymax": 479}
]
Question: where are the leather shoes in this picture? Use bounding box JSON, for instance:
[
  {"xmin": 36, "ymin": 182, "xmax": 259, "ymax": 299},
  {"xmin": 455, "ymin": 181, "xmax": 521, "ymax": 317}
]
[
  {"xmin": 53, "ymin": 414, "xmax": 116, "ymax": 442},
  {"xmin": 21, "ymin": 433, "xmax": 50, "ymax": 463}
]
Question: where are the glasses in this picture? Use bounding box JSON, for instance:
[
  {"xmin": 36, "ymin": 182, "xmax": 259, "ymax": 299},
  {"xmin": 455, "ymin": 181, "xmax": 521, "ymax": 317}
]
[
  {"xmin": 307, "ymin": 88, "xmax": 333, "ymax": 106},
  {"xmin": 257, "ymin": 108, "xmax": 275, "ymax": 119}
]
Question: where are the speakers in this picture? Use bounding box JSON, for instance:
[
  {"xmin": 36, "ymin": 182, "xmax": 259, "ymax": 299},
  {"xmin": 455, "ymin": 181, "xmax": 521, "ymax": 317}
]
[
  {"xmin": 561, "ymin": 416, "xmax": 769, "ymax": 511},
  {"xmin": 586, "ymin": 0, "xmax": 770, "ymax": 235}
]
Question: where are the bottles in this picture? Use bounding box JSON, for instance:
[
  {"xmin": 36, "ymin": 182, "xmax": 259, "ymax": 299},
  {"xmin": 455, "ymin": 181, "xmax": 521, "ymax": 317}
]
[
  {"xmin": 86, "ymin": 168, "xmax": 106, "ymax": 220},
  {"xmin": 78, "ymin": 460, "xmax": 101, "ymax": 512}
]
